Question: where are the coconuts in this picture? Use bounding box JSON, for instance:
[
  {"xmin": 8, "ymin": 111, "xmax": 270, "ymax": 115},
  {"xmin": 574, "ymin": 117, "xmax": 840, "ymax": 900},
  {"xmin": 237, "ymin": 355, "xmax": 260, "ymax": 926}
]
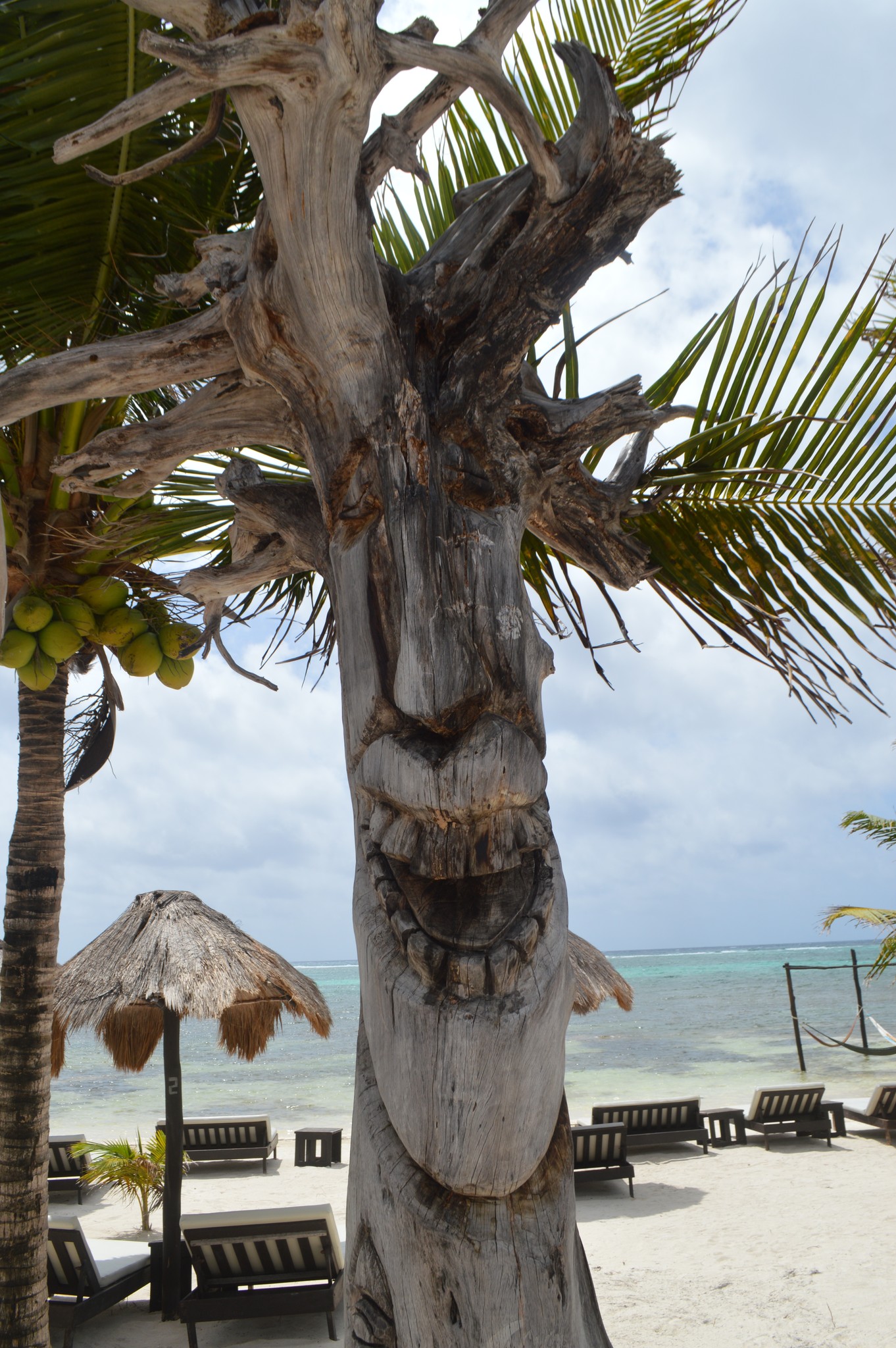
[{"xmin": 0, "ymin": 574, "xmax": 202, "ymax": 692}]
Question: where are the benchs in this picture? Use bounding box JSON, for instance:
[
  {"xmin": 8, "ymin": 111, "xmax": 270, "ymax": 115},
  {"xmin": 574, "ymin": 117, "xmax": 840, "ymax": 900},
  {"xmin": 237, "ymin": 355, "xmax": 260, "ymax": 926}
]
[
  {"xmin": 154, "ymin": 1110, "xmax": 283, "ymax": 1177},
  {"xmin": 730, "ymin": 1081, "xmax": 832, "ymax": 1152},
  {"xmin": 46, "ymin": 1131, "xmax": 97, "ymax": 1205},
  {"xmin": 174, "ymin": 1198, "xmax": 345, "ymax": 1348},
  {"xmin": 831, "ymin": 1080, "xmax": 896, "ymax": 1146},
  {"xmin": 569, "ymin": 1122, "xmax": 637, "ymax": 1202},
  {"xmin": 46, "ymin": 1213, "xmax": 152, "ymax": 1348},
  {"xmin": 575, "ymin": 1093, "xmax": 709, "ymax": 1156}
]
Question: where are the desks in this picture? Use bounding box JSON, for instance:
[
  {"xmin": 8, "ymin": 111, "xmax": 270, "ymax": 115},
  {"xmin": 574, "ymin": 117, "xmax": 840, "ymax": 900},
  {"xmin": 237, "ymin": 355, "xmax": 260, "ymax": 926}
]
[
  {"xmin": 699, "ymin": 1105, "xmax": 748, "ymax": 1149},
  {"xmin": 147, "ymin": 1239, "xmax": 193, "ymax": 1313},
  {"xmin": 820, "ymin": 1099, "xmax": 848, "ymax": 1139},
  {"xmin": 292, "ymin": 1123, "xmax": 345, "ymax": 1169}
]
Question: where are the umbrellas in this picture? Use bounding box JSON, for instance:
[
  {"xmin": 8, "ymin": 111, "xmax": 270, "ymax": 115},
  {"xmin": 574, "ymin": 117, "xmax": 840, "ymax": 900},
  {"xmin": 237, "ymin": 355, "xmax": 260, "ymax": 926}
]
[
  {"xmin": 52, "ymin": 890, "xmax": 333, "ymax": 1323},
  {"xmin": 565, "ymin": 930, "xmax": 633, "ymax": 1016}
]
